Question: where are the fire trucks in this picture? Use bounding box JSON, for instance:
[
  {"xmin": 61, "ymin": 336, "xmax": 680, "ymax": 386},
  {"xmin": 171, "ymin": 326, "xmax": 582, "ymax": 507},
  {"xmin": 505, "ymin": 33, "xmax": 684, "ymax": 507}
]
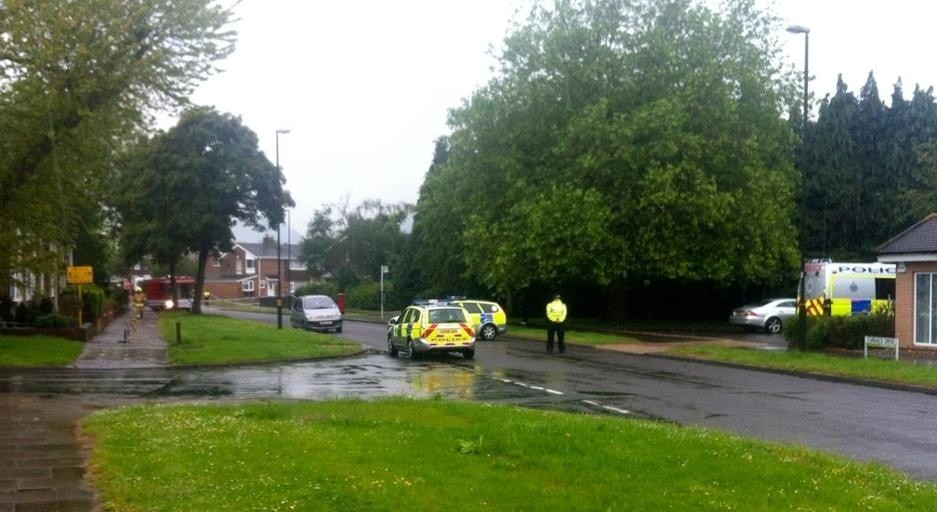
[{"xmin": 142, "ymin": 275, "xmax": 195, "ymax": 310}]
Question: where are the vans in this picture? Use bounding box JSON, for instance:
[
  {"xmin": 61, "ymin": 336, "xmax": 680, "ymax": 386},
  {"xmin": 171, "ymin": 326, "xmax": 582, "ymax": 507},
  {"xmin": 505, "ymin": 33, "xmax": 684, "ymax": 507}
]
[{"xmin": 800, "ymin": 258, "xmax": 898, "ymax": 318}]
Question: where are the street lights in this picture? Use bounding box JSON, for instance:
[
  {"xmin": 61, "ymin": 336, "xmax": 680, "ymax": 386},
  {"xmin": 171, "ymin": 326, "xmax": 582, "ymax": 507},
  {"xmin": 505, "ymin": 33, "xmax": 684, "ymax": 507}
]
[
  {"xmin": 276, "ymin": 129, "xmax": 290, "ymax": 330},
  {"xmin": 787, "ymin": 24, "xmax": 810, "ymax": 348}
]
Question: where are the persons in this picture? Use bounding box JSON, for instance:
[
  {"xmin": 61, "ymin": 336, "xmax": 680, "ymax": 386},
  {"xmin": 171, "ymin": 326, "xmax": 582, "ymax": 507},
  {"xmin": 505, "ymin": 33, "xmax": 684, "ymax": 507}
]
[
  {"xmin": 133, "ymin": 287, "xmax": 147, "ymax": 320},
  {"xmin": 546, "ymin": 295, "xmax": 568, "ymax": 353},
  {"xmin": 204, "ymin": 290, "xmax": 210, "ymax": 306}
]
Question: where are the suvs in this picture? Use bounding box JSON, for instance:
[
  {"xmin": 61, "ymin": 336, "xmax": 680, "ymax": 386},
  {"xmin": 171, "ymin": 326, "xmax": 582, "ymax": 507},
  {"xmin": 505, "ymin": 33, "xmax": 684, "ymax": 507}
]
[{"xmin": 386, "ymin": 297, "xmax": 508, "ymax": 361}]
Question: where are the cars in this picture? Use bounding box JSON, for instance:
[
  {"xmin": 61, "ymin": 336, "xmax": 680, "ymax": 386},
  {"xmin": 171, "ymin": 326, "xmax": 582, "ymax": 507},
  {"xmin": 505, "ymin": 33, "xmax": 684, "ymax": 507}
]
[
  {"xmin": 731, "ymin": 298, "xmax": 797, "ymax": 334},
  {"xmin": 292, "ymin": 295, "xmax": 343, "ymax": 333}
]
